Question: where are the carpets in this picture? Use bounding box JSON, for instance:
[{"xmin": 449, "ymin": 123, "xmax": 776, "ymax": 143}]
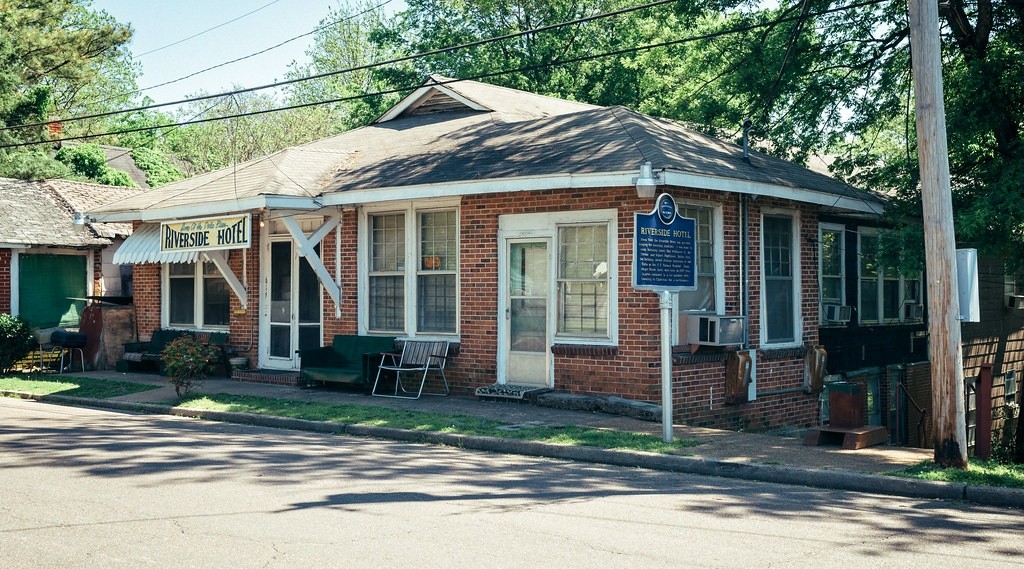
[{"xmin": 475, "ymin": 383, "xmax": 545, "ymax": 399}]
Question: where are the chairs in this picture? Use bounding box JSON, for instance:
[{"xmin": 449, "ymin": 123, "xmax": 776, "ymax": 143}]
[{"xmin": 372, "ymin": 339, "xmax": 450, "ymax": 399}]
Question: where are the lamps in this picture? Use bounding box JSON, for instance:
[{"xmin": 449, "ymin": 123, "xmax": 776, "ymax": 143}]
[
  {"xmin": 72, "ymin": 211, "xmax": 96, "ymax": 230},
  {"xmin": 633, "ymin": 161, "xmax": 662, "ymax": 198}
]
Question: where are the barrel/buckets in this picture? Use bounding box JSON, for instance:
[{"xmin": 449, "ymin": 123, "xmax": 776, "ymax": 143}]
[{"xmin": 228, "ymin": 357, "xmax": 248, "ymax": 375}]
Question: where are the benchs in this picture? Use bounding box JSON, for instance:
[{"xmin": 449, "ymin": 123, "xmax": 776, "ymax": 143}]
[
  {"xmin": 121, "ymin": 330, "xmax": 229, "ymax": 376},
  {"xmin": 295, "ymin": 335, "xmax": 396, "ymax": 395}
]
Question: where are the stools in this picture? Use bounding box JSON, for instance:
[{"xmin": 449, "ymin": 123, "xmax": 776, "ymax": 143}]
[
  {"xmin": 48, "ymin": 331, "xmax": 88, "ymax": 374},
  {"xmin": 30, "ymin": 327, "xmax": 66, "ymax": 374}
]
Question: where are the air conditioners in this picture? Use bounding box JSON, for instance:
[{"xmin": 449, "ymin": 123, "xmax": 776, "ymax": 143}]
[
  {"xmin": 1006, "ymin": 295, "xmax": 1024, "ymax": 309},
  {"xmin": 905, "ymin": 304, "xmax": 923, "ymax": 319},
  {"xmin": 823, "ymin": 304, "xmax": 851, "ymax": 322},
  {"xmin": 687, "ymin": 315, "xmax": 746, "ymax": 346}
]
